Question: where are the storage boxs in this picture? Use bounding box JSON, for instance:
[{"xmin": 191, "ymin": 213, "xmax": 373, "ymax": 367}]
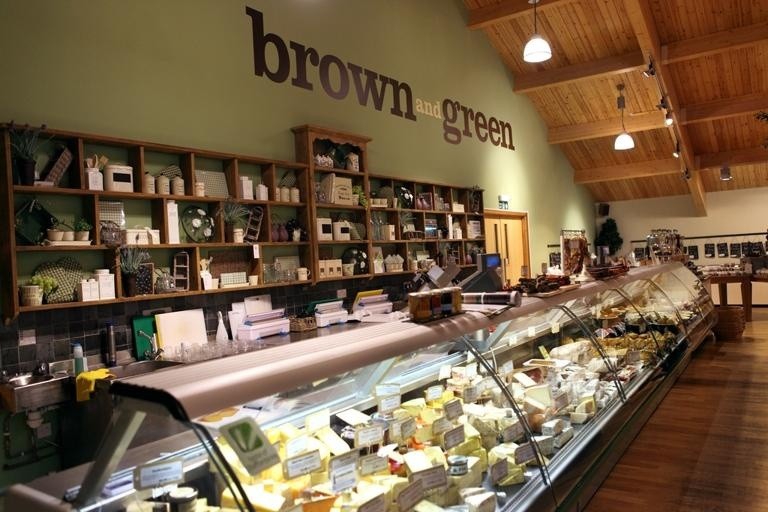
[
  {"xmin": 235, "ymin": 293, "xmax": 394, "ymax": 341},
  {"xmin": 103, "ymin": 228, "xmax": 160, "ymax": 245}
]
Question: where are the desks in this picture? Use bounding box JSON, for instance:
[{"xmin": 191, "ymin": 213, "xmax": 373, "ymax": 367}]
[{"xmin": 706, "ymin": 271, "xmax": 757, "ymax": 320}]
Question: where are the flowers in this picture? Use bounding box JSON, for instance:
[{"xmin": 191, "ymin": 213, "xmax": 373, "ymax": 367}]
[{"xmin": 111, "ymin": 243, "xmax": 150, "ymax": 275}]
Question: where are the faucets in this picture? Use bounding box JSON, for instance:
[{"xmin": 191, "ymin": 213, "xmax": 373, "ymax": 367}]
[
  {"xmin": 36, "ymin": 360, "xmax": 50, "ymax": 376},
  {"xmin": 137, "ymin": 329, "xmax": 164, "ymax": 358}
]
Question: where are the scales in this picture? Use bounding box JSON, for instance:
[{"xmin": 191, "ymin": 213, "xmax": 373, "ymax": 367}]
[{"xmin": 478, "ymin": 253, "xmax": 505, "ymax": 297}]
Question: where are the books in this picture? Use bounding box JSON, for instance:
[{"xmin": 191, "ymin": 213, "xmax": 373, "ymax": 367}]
[
  {"xmin": 460, "ymin": 290, "xmax": 522, "ymax": 319},
  {"xmin": 133, "ymin": 308, "xmax": 208, "ymax": 356}
]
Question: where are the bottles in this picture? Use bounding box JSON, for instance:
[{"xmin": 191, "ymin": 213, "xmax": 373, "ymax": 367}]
[
  {"xmin": 276, "ymin": 184, "xmax": 301, "ymax": 203},
  {"xmin": 84, "ymin": 168, "xmax": 105, "ymax": 191},
  {"xmin": 240, "ymin": 177, "xmax": 254, "ymax": 200},
  {"xmin": 155, "ymin": 272, "xmax": 176, "ymax": 292},
  {"xmin": 215, "ymin": 311, "xmax": 228, "ymax": 343},
  {"xmin": 255, "ymin": 184, "xmax": 268, "ymax": 199},
  {"xmin": 145, "ymin": 170, "xmax": 185, "ymax": 196},
  {"xmin": 71, "ymin": 341, "xmax": 85, "ymax": 374}
]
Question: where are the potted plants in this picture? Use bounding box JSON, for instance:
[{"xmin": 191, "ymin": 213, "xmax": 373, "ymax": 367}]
[
  {"xmin": 214, "ymin": 195, "xmax": 254, "ymax": 243},
  {"xmin": 17, "ymin": 277, "xmax": 58, "ymax": 306}
]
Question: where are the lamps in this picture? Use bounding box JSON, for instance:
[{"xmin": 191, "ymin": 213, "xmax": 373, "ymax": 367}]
[
  {"xmin": 720, "ymin": 167, "xmax": 732, "ymax": 183},
  {"xmin": 612, "ymin": 82, "xmax": 636, "ymax": 152},
  {"xmin": 644, "ymin": 53, "xmax": 691, "ymax": 180},
  {"xmin": 521, "ymin": 0, "xmax": 554, "ymax": 64}
]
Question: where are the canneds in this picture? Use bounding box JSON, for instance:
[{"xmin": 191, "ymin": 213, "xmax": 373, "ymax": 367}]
[{"xmin": 408, "ymin": 286, "xmax": 462, "ymax": 320}]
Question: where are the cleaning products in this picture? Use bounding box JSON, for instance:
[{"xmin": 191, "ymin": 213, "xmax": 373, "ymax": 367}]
[{"xmin": 71, "ymin": 338, "xmax": 84, "ymax": 377}]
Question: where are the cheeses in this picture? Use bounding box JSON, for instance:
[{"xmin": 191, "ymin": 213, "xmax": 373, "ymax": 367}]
[{"xmin": 122, "ymin": 331, "xmax": 675, "ymax": 512}]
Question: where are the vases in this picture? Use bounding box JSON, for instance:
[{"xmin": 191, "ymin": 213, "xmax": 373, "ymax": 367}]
[{"xmin": 122, "ymin": 270, "xmax": 137, "ymax": 298}]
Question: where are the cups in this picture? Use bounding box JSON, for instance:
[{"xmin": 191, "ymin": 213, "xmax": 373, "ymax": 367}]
[
  {"xmin": 248, "ymin": 276, "xmax": 259, "ymax": 285},
  {"xmin": 370, "ymin": 199, "xmax": 388, "ymax": 205},
  {"xmin": 196, "ymin": 182, "xmax": 204, "ymax": 197},
  {"xmin": 234, "ymin": 228, "xmax": 244, "ymax": 244},
  {"xmin": 21, "ymin": 286, "xmax": 43, "ymax": 305},
  {"xmin": 47, "ymin": 229, "xmax": 89, "ymax": 241},
  {"xmin": 297, "ymin": 267, "xmax": 311, "ymax": 281},
  {"xmin": 342, "ymin": 263, "xmax": 355, "ymax": 276}
]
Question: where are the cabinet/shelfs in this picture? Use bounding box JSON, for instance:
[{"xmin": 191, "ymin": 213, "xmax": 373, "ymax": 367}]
[
  {"xmin": 7, "ymin": 127, "xmax": 315, "ymax": 303},
  {"xmin": 461, "ymin": 259, "xmax": 717, "ymax": 512},
  {"xmin": 295, "ymin": 124, "xmax": 374, "ymax": 276},
  {"xmin": 68, "ymin": 299, "xmax": 559, "ymax": 511},
  {"xmin": 366, "ymin": 172, "xmax": 488, "ymax": 271}
]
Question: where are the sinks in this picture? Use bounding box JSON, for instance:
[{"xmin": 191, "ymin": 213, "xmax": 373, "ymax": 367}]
[
  {"xmin": 77, "ymin": 359, "xmax": 186, "ymax": 385},
  {"xmin": 0, "ymin": 370, "xmax": 71, "ymax": 415}
]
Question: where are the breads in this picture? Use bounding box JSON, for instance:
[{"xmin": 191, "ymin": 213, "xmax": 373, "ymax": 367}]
[{"xmin": 508, "ymin": 274, "xmax": 570, "ymax": 295}]
